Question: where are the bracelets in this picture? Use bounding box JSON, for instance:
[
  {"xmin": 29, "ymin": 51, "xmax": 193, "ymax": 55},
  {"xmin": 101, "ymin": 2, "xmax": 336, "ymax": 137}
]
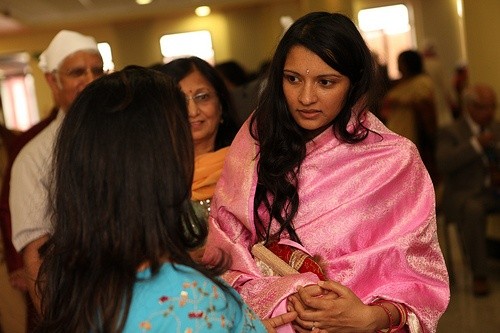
[
  {"xmin": 388, "ymin": 301, "xmax": 407, "ymax": 330},
  {"xmin": 367, "ymin": 301, "xmax": 393, "ymax": 333}
]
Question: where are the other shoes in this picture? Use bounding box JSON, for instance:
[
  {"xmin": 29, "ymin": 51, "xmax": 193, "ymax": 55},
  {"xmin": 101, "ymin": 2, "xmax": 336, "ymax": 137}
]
[{"xmin": 472, "ymin": 280, "xmax": 493, "ymax": 296}]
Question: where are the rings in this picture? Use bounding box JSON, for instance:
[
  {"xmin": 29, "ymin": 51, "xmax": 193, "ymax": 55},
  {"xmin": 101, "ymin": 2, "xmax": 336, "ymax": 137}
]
[{"xmin": 312, "ymin": 321, "xmax": 315, "ymax": 330}]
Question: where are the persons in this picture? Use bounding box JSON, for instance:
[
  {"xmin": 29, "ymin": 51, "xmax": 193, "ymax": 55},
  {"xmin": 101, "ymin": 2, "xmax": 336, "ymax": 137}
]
[
  {"xmin": 210, "ymin": 45, "xmax": 500, "ymax": 299},
  {"xmin": 194, "ymin": 12, "xmax": 450, "ymax": 333},
  {"xmin": 35, "ymin": 64, "xmax": 270, "ymax": 333},
  {"xmin": 9, "ymin": 30, "xmax": 105, "ymax": 316},
  {"xmin": 169, "ymin": 55, "xmax": 243, "ymax": 256}
]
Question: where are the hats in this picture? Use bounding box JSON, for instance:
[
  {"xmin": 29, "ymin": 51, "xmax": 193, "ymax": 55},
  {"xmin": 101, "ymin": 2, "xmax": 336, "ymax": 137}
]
[{"xmin": 38, "ymin": 29, "xmax": 99, "ymax": 73}]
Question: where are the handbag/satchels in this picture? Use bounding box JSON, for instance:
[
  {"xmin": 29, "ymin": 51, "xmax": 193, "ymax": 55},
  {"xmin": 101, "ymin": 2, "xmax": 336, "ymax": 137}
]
[
  {"xmin": 434, "ymin": 119, "xmax": 481, "ymax": 179},
  {"xmin": 249, "ymin": 235, "xmax": 327, "ymax": 284}
]
